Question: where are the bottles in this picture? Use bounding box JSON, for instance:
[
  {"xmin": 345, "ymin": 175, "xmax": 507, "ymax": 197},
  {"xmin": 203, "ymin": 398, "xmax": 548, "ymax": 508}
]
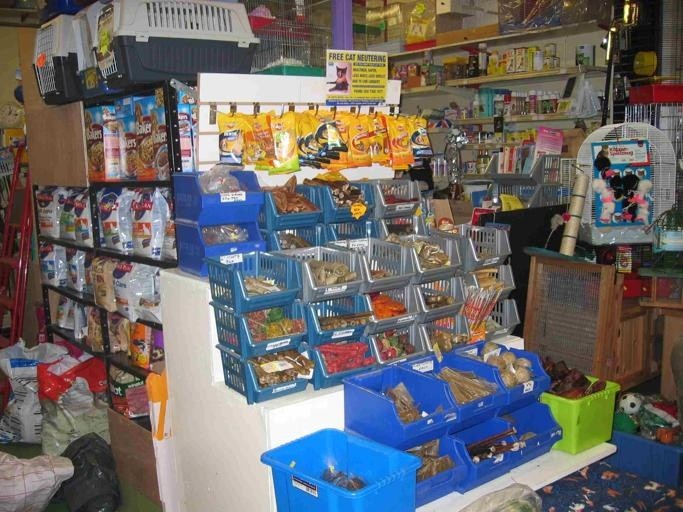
[
  {"xmin": 422, "ymin": 50, "xmax": 435, "ymax": 66},
  {"xmin": 471, "ymin": 87, "xmax": 511, "ymax": 118},
  {"xmin": 479, "ymin": 149, "xmax": 490, "ymax": 177},
  {"xmin": 476, "ymin": 42, "xmax": 488, "ymax": 75},
  {"xmin": 428, "ymin": 157, "xmax": 446, "ymax": 178},
  {"xmin": 528, "ymin": 89, "xmax": 559, "ymax": 115},
  {"xmin": 459, "ymin": 483, "xmax": 542, "ymax": 511}
]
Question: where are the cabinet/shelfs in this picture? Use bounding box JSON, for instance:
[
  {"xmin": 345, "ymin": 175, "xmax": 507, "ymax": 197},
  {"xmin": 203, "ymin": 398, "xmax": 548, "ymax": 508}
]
[
  {"xmin": 33, "ymin": 181, "xmax": 179, "ymax": 433},
  {"xmin": 388, "ymin": 19, "xmax": 608, "ymax": 177},
  {"xmin": 610, "ymin": 428, "xmax": 683, "ymax": 488}
]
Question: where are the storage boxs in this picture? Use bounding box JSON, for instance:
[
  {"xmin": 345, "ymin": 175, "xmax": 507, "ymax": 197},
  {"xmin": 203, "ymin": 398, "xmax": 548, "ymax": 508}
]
[
  {"xmin": 260, "ymin": 428, "xmax": 423, "ymax": 512},
  {"xmin": 539, "ymin": 375, "xmax": 621, "ymax": 455}
]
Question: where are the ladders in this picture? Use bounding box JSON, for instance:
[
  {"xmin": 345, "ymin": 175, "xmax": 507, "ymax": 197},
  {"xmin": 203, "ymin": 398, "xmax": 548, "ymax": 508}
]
[{"xmin": 0, "ymin": 146, "xmax": 33, "ymax": 411}]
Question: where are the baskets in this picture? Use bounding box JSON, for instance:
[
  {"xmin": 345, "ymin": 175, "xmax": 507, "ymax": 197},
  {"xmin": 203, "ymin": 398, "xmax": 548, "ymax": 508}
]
[{"xmin": 203, "ymin": 178, "xmax": 521, "ymax": 404}]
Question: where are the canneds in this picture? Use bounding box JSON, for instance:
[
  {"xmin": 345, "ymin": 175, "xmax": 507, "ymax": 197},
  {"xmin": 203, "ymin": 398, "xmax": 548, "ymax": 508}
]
[{"xmin": 533, "ymin": 43, "xmax": 559, "ymax": 70}]
